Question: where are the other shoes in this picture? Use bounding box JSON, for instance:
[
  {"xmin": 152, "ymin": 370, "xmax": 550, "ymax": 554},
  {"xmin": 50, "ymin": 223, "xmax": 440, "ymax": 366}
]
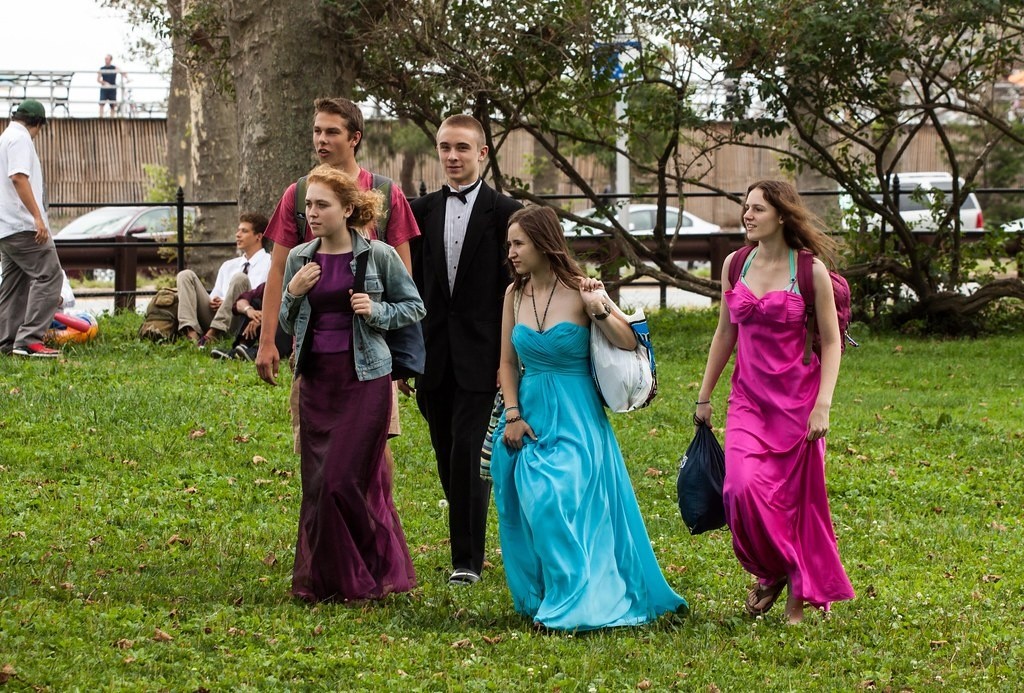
[
  {"xmin": 533, "ymin": 622, "xmax": 547, "ymax": 634},
  {"xmin": 235, "ymin": 346, "xmax": 258, "ymax": 363},
  {"xmin": 449, "ymin": 567, "xmax": 483, "ymax": 586},
  {"xmin": 186, "ymin": 335, "xmax": 201, "ymax": 344},
  {"xmin": 197, "ymin": 336, "xmax": 212, "ymax": 348},
  {"xmin": 211, "ymin": 349, "xmax": 236, "ymax": 360}
]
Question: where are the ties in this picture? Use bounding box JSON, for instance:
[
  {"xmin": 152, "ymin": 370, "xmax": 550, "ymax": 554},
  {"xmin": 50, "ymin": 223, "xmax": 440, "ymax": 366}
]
[{"xmin": 243, "ymin": 262, "xmax": 250, "ymax": 275}]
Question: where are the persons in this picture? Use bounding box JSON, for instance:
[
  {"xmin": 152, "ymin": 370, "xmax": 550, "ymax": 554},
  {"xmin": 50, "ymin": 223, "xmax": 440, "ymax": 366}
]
[
  {"xmin": 210, "ymin": 246, "xmax": 294, "ymax": 362},
  {"xmin": 694, "ymin": 179, "xmax": 855, "ymax": 625},
  {"xmin": 0, "ymin": 100, "xmax": 64, "ymax": 358},
  {"xmin": 254, "ymin": 99, "xmax": 421, "ymax": 477},
  {"xmin": 175, "ymin": 213, "xmax": 270, "ymax": 348},
  {"xmin": 489, "ymin": 204, "xmax": 688, "ymax": 630},
  {"xmin": 405, "ymin": 113, "xmax": 526, "ymax": 585},
  {"xmin": 279, "ymin": 162, "xmax": 427, "ymax": 609},
  {"xmin": 97, "ymin": 54, "xmax": 129, "ymax": 118},
  {"xmin": 51, "ymin": 268, "xmax": 75, "ymax": 331}
]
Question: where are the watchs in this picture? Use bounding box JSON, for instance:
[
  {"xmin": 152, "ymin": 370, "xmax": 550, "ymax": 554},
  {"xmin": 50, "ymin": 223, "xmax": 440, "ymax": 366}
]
[{"xmin": 592, "ymin": 304, "xmax": 611, "ymax": 320}]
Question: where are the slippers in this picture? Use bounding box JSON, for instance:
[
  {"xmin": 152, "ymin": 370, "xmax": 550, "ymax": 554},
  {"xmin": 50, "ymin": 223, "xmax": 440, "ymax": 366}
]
[
  {"xmin": 779, "ymin": 641, "xmax": 804, "ymax": 648},
  {"xmin": 745, "ymin": 574, "xmax": 787, "ymax": 616}
]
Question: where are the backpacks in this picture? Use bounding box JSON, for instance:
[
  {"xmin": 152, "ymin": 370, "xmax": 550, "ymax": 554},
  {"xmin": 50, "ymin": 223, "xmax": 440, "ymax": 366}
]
[
  {"xmin": 728, "ymin": 245, "xmax": 859, "ymax": 365},
  {"xmin": 138, "ymin": 287, "xmax": 183, "ymax": 346}
]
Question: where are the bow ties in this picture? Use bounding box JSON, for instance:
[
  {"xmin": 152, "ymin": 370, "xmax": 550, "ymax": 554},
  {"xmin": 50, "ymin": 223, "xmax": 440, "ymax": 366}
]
[{"xmin": 442, "ymin": 178, "xmax": 480, "ymax": 204}]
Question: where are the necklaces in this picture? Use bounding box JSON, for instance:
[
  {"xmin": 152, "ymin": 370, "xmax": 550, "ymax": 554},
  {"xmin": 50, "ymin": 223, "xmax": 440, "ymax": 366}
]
[{"xmin": 531, "ymin": 278, "xmax": 558, "ymax": 334}]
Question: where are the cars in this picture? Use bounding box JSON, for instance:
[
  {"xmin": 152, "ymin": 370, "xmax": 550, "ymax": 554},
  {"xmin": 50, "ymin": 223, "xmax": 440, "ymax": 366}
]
[
  {"xmin": 559, "ymin": 203, "xmax": 722, "ymax": 274},
  {"xmin": 53, "ymin": 206, "xmax": 201, "ymax": 283}
]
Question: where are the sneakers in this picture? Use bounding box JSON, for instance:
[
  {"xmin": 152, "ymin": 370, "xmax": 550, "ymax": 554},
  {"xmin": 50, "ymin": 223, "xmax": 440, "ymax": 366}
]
[{"xmin": 12, "ymin": 343, "xmax": 63, "ymax": 357}]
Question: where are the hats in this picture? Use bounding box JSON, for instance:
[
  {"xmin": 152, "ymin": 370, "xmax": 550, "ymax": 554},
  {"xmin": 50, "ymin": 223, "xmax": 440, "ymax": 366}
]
[{"xmin": 15, "ymin": 100, "xmax": 47, "ymax": 126}]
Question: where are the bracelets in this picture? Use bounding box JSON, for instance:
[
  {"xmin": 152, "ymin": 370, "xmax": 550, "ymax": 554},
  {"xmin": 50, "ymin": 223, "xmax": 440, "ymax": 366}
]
[
  {"xmin": 505, "ymin": 416, "xmax": 521, "ymax": 423},
  {"xmin": 696, "ymin": 401, "xmax": 710, "ymax": 404},
  {"xmin": 505, "ymin": 407, "xmax": 519, "ymax": 412},
  {"xmin": 244, "ymin": 306, "xmax": 253, "ymax": 315}
]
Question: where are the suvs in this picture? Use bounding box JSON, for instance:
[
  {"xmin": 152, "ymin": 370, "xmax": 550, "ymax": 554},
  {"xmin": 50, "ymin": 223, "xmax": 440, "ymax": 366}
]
[{"xmin": 839, "ymin": 171, "xmax": 982, "ymax": 230}]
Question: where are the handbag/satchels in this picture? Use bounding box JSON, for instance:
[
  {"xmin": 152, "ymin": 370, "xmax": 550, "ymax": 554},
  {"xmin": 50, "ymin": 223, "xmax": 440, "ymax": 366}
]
[
  {"xmin": 478, "ymin": 368, "xmax": 526, "ymax": 481},
  {"xmin": 676, "ymin": 412, "xmax": 730, "ymax": 536},
  {"xmin": 354, "ymin": 237, "xmax": 426, "ymax": 376},
  {"xmin": 590, "ymin": 289, "xmax": 657, "ymax": 414}
]
